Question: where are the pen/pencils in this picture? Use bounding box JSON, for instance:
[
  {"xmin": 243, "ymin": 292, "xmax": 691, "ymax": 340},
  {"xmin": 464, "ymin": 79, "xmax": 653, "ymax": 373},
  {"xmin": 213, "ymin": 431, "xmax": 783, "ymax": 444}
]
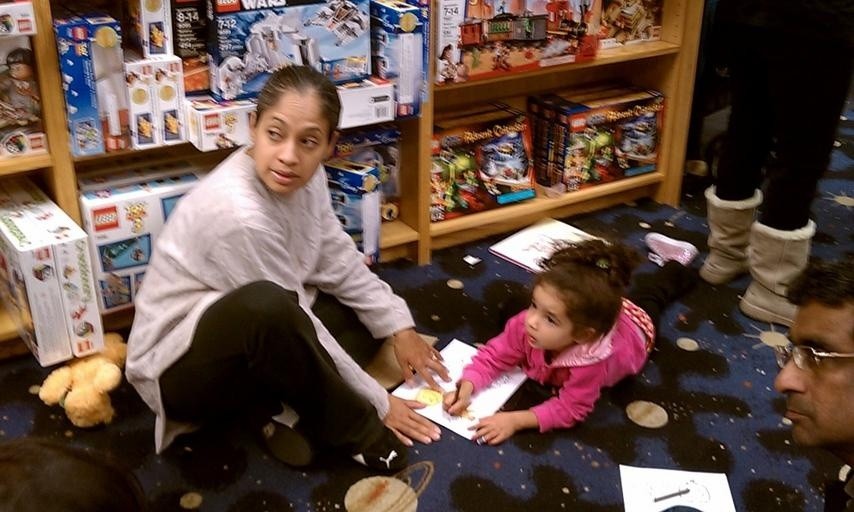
[
  {"xmin": 654, "ymin": 489, "xmax": 690, "ymax": 502},
  {"xmin": 456, "ymin": 382, "xmax": 461, "ymax": 401}
]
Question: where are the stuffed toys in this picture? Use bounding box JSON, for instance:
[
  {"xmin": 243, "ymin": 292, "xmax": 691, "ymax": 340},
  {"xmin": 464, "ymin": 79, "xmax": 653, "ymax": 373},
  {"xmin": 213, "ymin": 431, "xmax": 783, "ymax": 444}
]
[{"xmin": 36, "ymin": 328, "xmax": 129, "ymax": 427}]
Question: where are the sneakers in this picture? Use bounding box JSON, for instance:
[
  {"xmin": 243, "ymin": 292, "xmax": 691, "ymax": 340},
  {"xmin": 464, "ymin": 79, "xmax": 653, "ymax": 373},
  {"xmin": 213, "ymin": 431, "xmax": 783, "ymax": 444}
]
[
  {"xmin": 360, "ymin": 428, "xmax": 408, "ymax": 473},
  {"xmin": 645, "ymin": 232, "xmax": 698, "ymax": 265},
  {"xmin": 255, "ymin": 417, "xmax": 313, "ymax": 467}
]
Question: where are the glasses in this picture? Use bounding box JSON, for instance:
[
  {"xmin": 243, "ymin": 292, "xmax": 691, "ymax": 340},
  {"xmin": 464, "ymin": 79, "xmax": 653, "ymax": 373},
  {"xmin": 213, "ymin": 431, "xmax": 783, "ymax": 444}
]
[{"xmin": 773, "ymin": 341, "xmax": 854, "ymax": 370}]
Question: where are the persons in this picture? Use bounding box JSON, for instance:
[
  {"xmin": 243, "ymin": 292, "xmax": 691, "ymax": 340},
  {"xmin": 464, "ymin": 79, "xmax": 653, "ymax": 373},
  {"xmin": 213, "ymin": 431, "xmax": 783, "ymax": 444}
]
[
  {"xmin": 697, "ymin": 0, "xmax": 852, "ymax": 330},
  {"xmin": 771, "ymin": 253, "xmax": 854, "ymax": 512},
  {"xmin": 438, "ymin": 231, "xmax": 700, "ymax": 447},
  {"xmin": 123, "ymin": 63, "xmax": 453, "ymax": 472}
]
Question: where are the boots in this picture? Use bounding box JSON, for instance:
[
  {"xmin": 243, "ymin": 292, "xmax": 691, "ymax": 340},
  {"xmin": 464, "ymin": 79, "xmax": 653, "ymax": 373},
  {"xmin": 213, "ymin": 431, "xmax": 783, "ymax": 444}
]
[
  {"xmin": 739, "ymin": 220, "xmax": 816, "ymax": 328},
  {"xmin": 699, "ymin": 186, "xmax": 762, "ymax": 286}
]
